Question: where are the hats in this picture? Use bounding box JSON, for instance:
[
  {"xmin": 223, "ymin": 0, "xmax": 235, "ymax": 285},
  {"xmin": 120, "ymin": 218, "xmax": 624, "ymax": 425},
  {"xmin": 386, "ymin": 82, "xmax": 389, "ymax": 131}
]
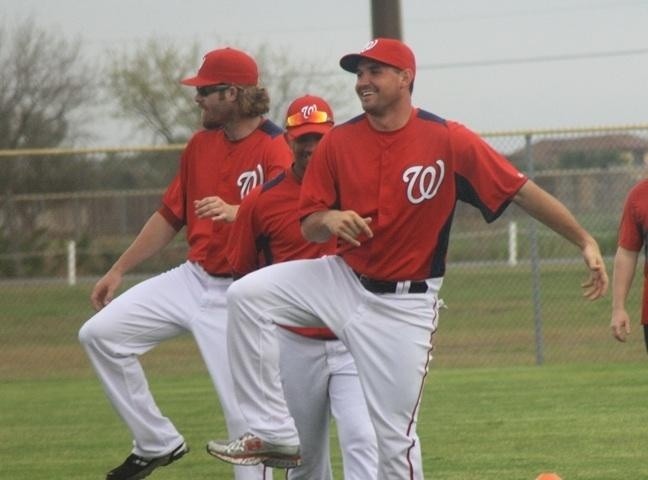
[
  {"xmin": 286, "ymin": 95, "xmax": 334, "ymax": 141},
  {"xmin": 182, "ymin": 48, "xmax": 258, "ymax": 87},
  {"xmin": 340, "ymin": 38, "xmax": 415, "ymax": 81}
]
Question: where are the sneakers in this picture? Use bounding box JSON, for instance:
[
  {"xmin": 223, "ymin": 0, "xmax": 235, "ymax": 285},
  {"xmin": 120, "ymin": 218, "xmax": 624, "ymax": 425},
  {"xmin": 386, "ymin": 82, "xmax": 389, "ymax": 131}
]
[
  {"xmin": 207, "ymin": 433, "xmax": 302, "ymax": 467},
  {"xmin": 107, "ymin": 441, "xmax": 189, "ymax": 479}
]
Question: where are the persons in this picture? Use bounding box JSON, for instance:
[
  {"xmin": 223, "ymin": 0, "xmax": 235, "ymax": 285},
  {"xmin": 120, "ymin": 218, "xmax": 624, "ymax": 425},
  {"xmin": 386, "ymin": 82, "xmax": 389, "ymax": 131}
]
[
  {"xmin": 207, "ymin": 37, "xmax": 607, "ymax": 480},
  {"xmin": 224, "ymin": 94, "xmax": 378, "ymax": 480},
  {"xmin": 610, "ymin": 179, "xmax": 648, "ymax": 354},
  {"xmin": 77, "ymin": 48, "xmax": 292, "ymax": 479}
]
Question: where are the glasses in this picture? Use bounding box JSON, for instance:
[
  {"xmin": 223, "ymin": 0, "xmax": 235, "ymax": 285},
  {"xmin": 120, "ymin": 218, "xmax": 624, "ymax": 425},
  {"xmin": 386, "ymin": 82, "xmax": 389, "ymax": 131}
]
[
  {"xmin": 287, "ymin": 111, "xmax": 332, "ymax": 126},
  {"xmin": 197, "ymin": 85, "xmax": 229, "ymax": 97}
]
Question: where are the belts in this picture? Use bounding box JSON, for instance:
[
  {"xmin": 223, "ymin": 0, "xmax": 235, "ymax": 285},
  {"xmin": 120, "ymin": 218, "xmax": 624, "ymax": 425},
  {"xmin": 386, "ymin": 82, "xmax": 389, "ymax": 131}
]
[{"xmin": 352, "ymin": 266, "xmax": 427, "ymax": 293}]
